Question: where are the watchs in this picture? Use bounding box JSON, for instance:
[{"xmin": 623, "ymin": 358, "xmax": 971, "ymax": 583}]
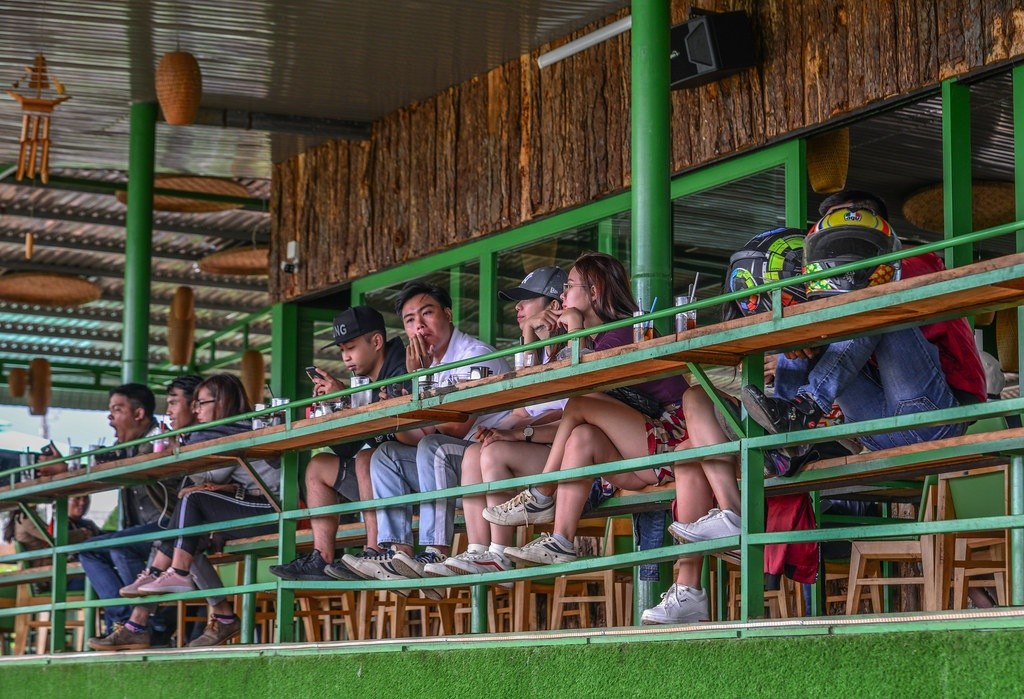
[{"xmin": 523, "ymin": 425, "xmax": 534, "ymax": 442}]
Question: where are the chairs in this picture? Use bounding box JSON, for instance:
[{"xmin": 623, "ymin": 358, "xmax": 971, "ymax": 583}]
[{"xmin": 0, "ymin": 474, "xmax": 1024, "ymax": 656}]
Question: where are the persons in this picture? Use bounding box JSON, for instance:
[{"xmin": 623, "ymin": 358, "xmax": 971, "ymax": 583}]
[
  {"xmin": 39, "ymin": 383, "xmax": 194, "ymax": 647},
  {"xmin": 87, "ymin": 374, "xmax": 241, "ymax": 651},
  {"xmin": 268, "ymin": 305, "xmax": 413, "ymax": 581},
  {"xmin": 423, "ymin": 266, "xmax": 595, "ymax": 590},
  {"xmin": 342, "ymin": 280, "xmax": 513, "ymax": 600},
  {"xmin": 0, "ymin": 494, "xmax": 104, "ymax": 591},
  {"xmin": 711, "ymin": 189, "xmax": 988, "ymax": 476},
  {"xmin": 483, "ymin": 250, "xmax": 688, "ymax": 564},
  {"xmin": 120, "ymin": 373, "xmax": 282, "ymax": 595},
  {"xmin": 642, "ymin": 351, "xmax": 780, "ymax": 625}
]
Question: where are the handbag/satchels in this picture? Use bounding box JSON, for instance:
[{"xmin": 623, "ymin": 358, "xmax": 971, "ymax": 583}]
[
  {"xmin": 186, "ymin": 416, "xmax": 251, "ymax": 446},
  {"xmin": 605, "ymin": 385, "xmax": 664, "ymax": 421}
]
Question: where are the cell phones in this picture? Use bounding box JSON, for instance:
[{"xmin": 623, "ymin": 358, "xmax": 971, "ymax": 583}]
[
  {"xmin": 41, "ymin": 444, "xmax": 54, "ymax": 456},
  {"xmin": 304, "ymin": 367, "xmax": 325, "ymax": 386}
]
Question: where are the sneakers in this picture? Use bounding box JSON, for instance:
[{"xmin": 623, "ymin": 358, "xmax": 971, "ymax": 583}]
[
  {"xmin": 424, "ymin": 551, "xmax": 473, "ymax": 587},
  {"xmin": 640, "ymin": 583, "xmax": 710, "ymax": 624},
  {"xmin": 341, "ymin": 546, "xmax": 412, "ymax": 599},
  {"xmin": 391, "ymin": 550, "xmax": 447, "ymax": 601},
  {"xmin": 482, "ymin": 490, "xmax": 555, "ymax": 528},
  {"xmin": 503, "ymin": 532, "xmax": 577, "ymax": 566},
  {"xmin": 87, "ymin": 620, "xmax": 152, "ymax": 650},
  {"xmin": 667, "ymin": 508, "xmax": 743, "ymax": 567},
  {"xmin": 741, "ymin": 385, "xmax": 821, "ymax": 459},
  {"xmin": 137, "ymin": 567, "xmax": 196, "ymax": 594},
  {"xmin": 269, "ymin": 549, "xmax": 338, "ymax": 581},
  {"xmin": 324, "ymin": 551, "xmax": 370, "ymax": 581},
  {"xmin": 712, "ymin": 398, "xmax": 818, "ymax": 477},
  {"xmin": 444, "ymin": 550, "xmax": 516, "ymax": 591},
  {"xmin": 189, "ymin": 613, "xmax": 241, "ymax": 647},
  {"xmin": 118, "ymin": 568, "xmax": 160, "ymax": 597}
]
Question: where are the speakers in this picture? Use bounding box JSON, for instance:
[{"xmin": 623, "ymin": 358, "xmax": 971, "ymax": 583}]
[{"xmin": 667, "ymin": 9, "xmax": 757, "ymax": 89}]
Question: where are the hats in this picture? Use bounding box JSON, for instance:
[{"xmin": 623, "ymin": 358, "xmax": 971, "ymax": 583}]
[
  {"xmin": 320, "ymin": 306, "xmax": 385, "ymax": 350},
  {"xmin": 498, "ymin": 266, "xmax": 567, "ymax": 302}
]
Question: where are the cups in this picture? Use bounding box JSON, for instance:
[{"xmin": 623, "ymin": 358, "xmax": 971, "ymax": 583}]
[
  {"xmin": 412, "ymin": 363, "xmax": 455, "ymax": 391},
  {"xmin": 351, "ymin": 376, "xmax": 372, "ymax": 411},
  {"xmin": 252, "ymin": 397, "xmax": 290, "ymax": 430},
  {"xmin": 675, "ymin": 296, "xmax": 696, "ymax": 333},
  {"xmin": 88, "ymin": 444, "xmax": 102, "ymax": 467},
  {"xmin": 514, "ymin": 352, "xmax": 524, "ymax": 371},
  {"xmin": 632, "ymin": 311, "xmax": 653, "ymax": 343},
  {"xmin": 19, "ymin": 454, "xmax": 35, "ymax": 482},
  {"xmin": 153, "ymin": 427, "xmax": 170, "ymax": 454},
  {"xmin": 67, "ymin": 447, "xmax": 82, "ymax": 471}
]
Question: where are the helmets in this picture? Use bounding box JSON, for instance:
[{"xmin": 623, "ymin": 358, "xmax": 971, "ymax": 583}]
[
  {"xmin": 978, "ymin": 349, "xmax": 1004, "ymax": 399},
  {"xmin": 728, "ymin": 228, "xmax": 808, "ymax": 316},
  {"xmin": 802, "ymin": 208, "xmax": 902, "ymax": 301}
]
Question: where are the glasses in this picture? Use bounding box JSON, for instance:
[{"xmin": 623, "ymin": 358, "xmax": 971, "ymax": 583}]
[
  {"xmin": 562, "ymin": 282, "xmax": 593, "ymax": 293},
  {"xmin": 191, "ymin": 400, "xmax": 215, "ymax": 414}
]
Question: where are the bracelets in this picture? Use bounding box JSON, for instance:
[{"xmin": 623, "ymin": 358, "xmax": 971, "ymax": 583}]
[{"xmin": 568, "ymin": 328, "xmax": 585, "ymax": 333}]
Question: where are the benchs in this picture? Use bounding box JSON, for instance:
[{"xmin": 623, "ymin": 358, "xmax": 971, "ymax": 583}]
[{"xmin": 0, "ymin": 427, "xmax": 1024, "ymax": 646}]
